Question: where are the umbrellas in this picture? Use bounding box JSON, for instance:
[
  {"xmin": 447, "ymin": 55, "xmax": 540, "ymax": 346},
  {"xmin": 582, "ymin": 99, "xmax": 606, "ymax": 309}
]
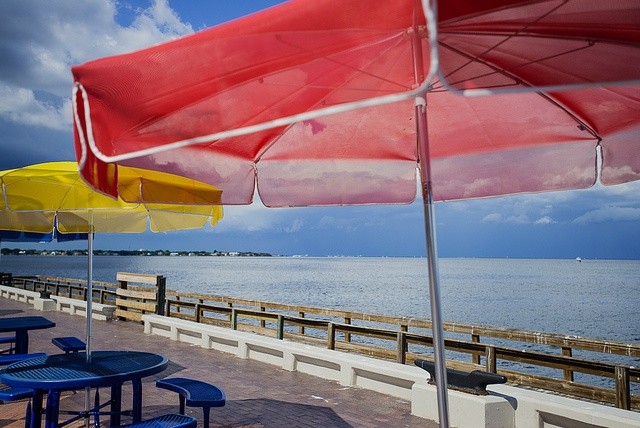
[
  {"xmin": 0, "ymin": 161, "xmax": 224, "ymax": 427},
  {"xmin": 0, "ymin": 194, "xmax": 95, "ymax": 243},
  {"xmin": 71, "ymin": 0, "xmax": 640, "ymax": 427}
]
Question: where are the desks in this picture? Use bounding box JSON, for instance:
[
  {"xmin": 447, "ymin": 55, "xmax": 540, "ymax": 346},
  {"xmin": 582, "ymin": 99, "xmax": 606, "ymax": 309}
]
[
  {"xmin": 0, "ymin": 349, "xmax": 168, "ymax": 428},
  {"xmin": 1, "ymin": 315, "xmax": 57, "ymax": 357}
]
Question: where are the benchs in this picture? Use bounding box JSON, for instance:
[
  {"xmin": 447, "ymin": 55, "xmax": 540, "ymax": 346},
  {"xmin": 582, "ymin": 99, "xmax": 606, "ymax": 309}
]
[
  {"xmin": 0, "ymin": 335, "xmax": 16, "ymax": 352},
  {"xmin": 50, "ymin": 335, "xmax": 86, "ymax": 352},
  {"xmin": 155, "ymin": 375, "xmax": 227, "ymax": 427},
  {"xmin": 112, "ymin": 414, "xmax": 199, "ymax": 427},
  {"xmin": 1, "ymin": 351, "xmax": 46, "ymax": 364},
  {"xmin": 0, "ymin": 387, "xmax": 38, "ymax": 427}
]
